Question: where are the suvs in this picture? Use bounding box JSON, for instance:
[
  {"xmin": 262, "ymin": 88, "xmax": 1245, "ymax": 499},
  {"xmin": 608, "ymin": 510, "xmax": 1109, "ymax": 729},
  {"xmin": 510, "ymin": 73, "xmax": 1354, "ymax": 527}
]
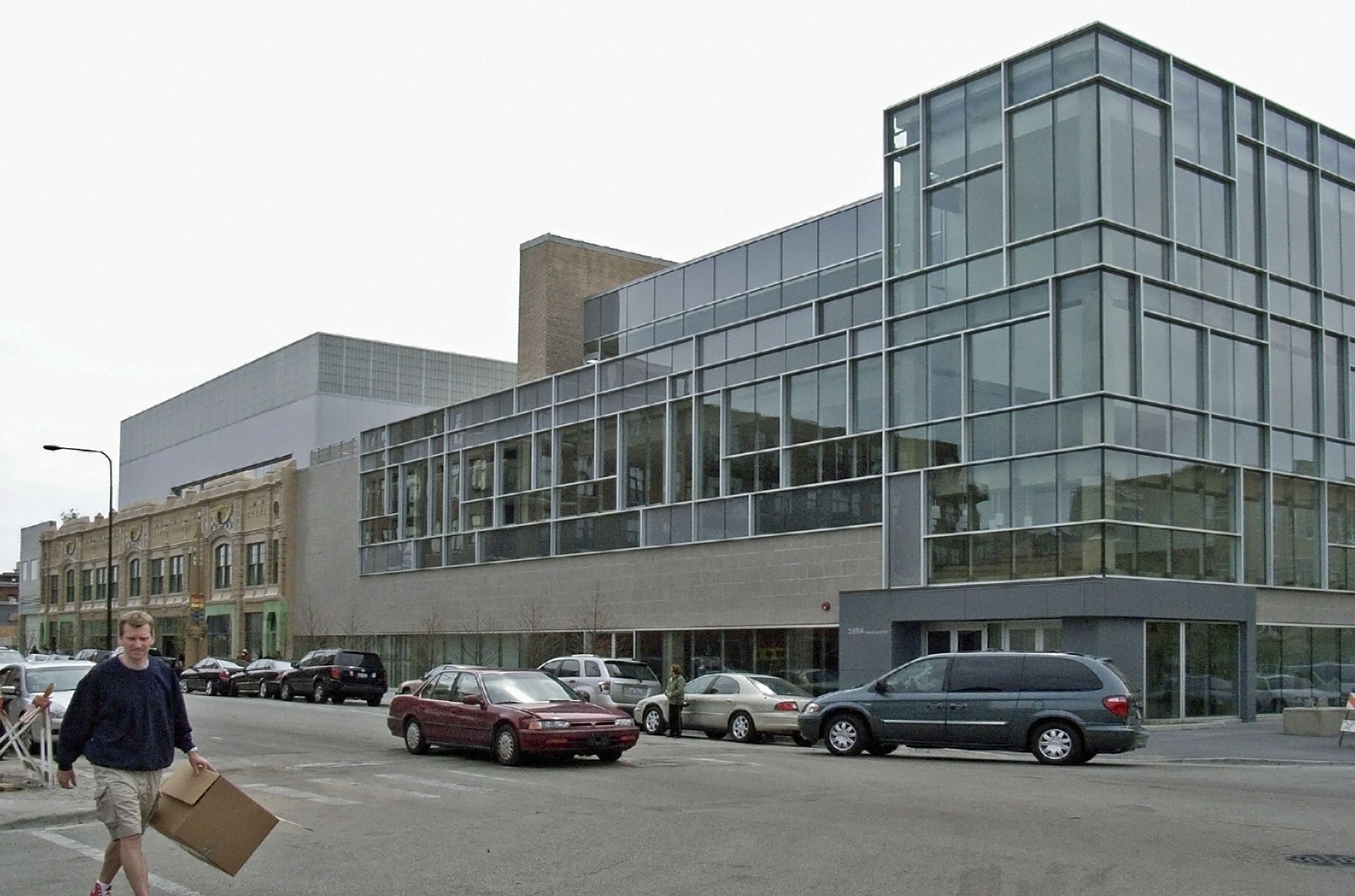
[
  {"xmin": 280, "ymin": 647, "xmax": 388, "ymax": 707},
  {"xmin": 536, "ymin": 654, "xmax": 661, "ymax": 717},
  {"xmin": 798, "ymin": 649, "xmax": 1149, "ymax": 765}
]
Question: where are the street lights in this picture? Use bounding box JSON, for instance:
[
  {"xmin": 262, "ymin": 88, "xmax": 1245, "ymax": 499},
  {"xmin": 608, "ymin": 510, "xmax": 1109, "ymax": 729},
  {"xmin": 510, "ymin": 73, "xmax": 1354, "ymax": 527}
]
[{"xmin": 42, "ymin": 443, "xmax": 113, "ymax": 652}]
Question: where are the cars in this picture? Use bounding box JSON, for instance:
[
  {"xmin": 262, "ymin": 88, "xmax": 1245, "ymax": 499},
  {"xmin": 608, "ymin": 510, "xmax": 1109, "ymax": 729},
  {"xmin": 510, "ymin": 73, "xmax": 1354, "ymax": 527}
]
[
  {"xmin": 228, "ymin": 658, "xmax": 299, "ymax": 699},
  {"xmin": 177, "ymin": 657, "xmax": 251, "ymax": 696},
  {"xmin": 394, "ymin": 663, "xmax": 489, "ymax": 696},
  {"xmin": 1148, "ymin": 659, "xmax": 1355, "ymax": 709},
  {"xmin": 386, "ymin": 667, "xmax": 641, "ymax": 767},
  {"xmin": 770, "ymin": 669, "xmax": 840, "ymax": 696},
  {"xmin": 692, "ymin": 656, "xmax": 748, "ymax": 675},
  {"xmin": 632, "ymin": 673, "xmax": 818, "ymax": 748},
  {"xmin": 1, "ymin": 645, "xmax": 160, "ymax": 756}
]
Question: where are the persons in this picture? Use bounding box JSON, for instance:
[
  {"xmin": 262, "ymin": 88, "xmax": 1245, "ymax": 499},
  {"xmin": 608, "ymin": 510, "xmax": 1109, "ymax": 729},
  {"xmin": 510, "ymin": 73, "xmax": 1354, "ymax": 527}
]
[
  {"xmin": 664, "ymin": 664, "xmax": 686, "ymax": 738},
  {"xmin": 236, "ymin": 648, "xmax": 250, "ymax": 663},
  {"xmin": 53, "ymin": 610, "xmax": 217, "ymax": 895}
]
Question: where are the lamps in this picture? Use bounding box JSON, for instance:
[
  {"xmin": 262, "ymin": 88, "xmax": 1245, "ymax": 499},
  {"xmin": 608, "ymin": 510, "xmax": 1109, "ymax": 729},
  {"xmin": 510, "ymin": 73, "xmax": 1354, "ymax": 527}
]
[{"xmin": 171, "ymin": 487, "xmax": 181, "ymax": 497}]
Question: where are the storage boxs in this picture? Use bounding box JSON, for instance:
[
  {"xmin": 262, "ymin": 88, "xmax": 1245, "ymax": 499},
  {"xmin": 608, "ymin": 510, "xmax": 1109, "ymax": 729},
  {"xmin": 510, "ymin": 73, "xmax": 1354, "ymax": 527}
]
[{"xmin": 149, "ymin": 761, "xmax": 279, "ymax": 876}]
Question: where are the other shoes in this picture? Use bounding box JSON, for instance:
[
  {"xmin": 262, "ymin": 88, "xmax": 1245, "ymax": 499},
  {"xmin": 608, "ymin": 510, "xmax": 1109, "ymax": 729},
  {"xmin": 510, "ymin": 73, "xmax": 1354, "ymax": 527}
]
[{"xmin": 90, "ymin": 880, "xmax": 113, "ymax": 896}]
